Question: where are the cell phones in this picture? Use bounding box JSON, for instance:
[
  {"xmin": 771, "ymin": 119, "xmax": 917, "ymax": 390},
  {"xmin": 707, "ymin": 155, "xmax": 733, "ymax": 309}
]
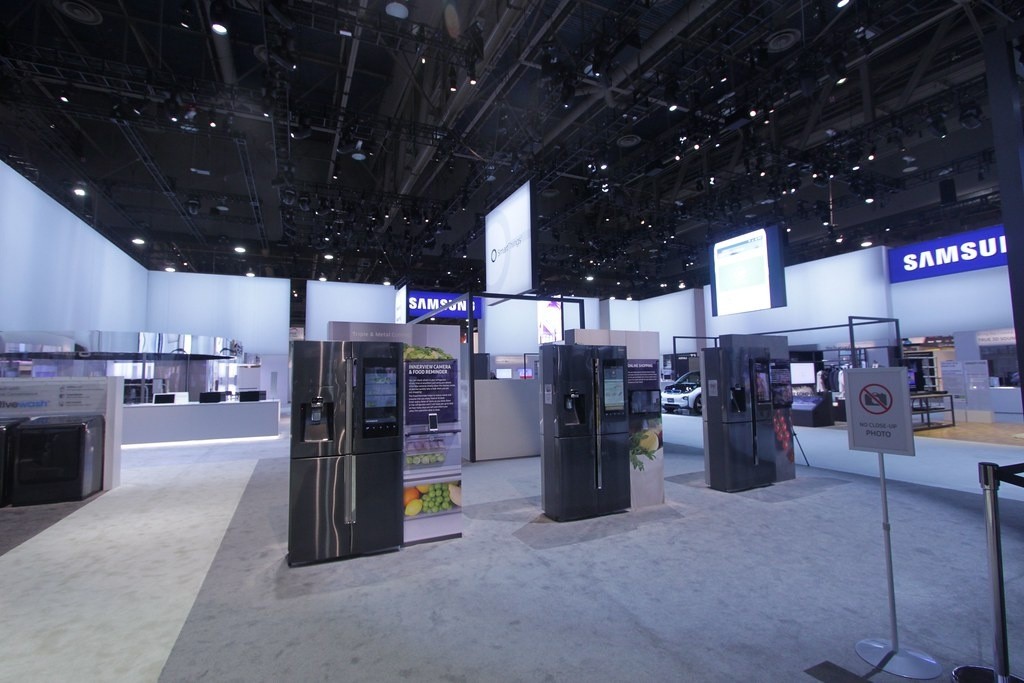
[{"xmin": 428, "ymin": 413, "xmax": 438, "ymax": 431}]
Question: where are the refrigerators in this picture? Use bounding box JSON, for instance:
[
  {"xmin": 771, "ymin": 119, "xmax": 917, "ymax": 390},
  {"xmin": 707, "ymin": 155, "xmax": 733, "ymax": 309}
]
[
  {"xmin": 700, "ymin": 346, "xmax": 776, "ymax": 493},
  {"xmin": 539, "ymin": 344, "xmax": 631, "ymax": 521},
  {"xmin": 285, "ymin": 339, "xmax": 405, "ymax": 566}
]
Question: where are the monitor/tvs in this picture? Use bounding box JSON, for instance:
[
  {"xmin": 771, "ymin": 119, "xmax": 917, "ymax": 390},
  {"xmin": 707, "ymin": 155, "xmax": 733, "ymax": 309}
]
[
  {"xmin": 757, "ymin": 370, "xmax": 770, "ymax": 402},
  {"xmin": 364, "ymin": 368, "xmax": 397, "ymax": 409},
  {"xmin": 629, "ymin": 389, "xmax": 661, "ymax": 414},
  {"xmin": 604, "ymin": 366, "xmax": 624, "ymax": 410}
]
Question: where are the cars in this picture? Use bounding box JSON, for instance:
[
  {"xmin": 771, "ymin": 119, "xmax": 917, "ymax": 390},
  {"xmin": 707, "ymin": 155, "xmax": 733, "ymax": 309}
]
[{"xmin": 662, "ymin": 371, "xmax": 703, "ymax": 416}]
